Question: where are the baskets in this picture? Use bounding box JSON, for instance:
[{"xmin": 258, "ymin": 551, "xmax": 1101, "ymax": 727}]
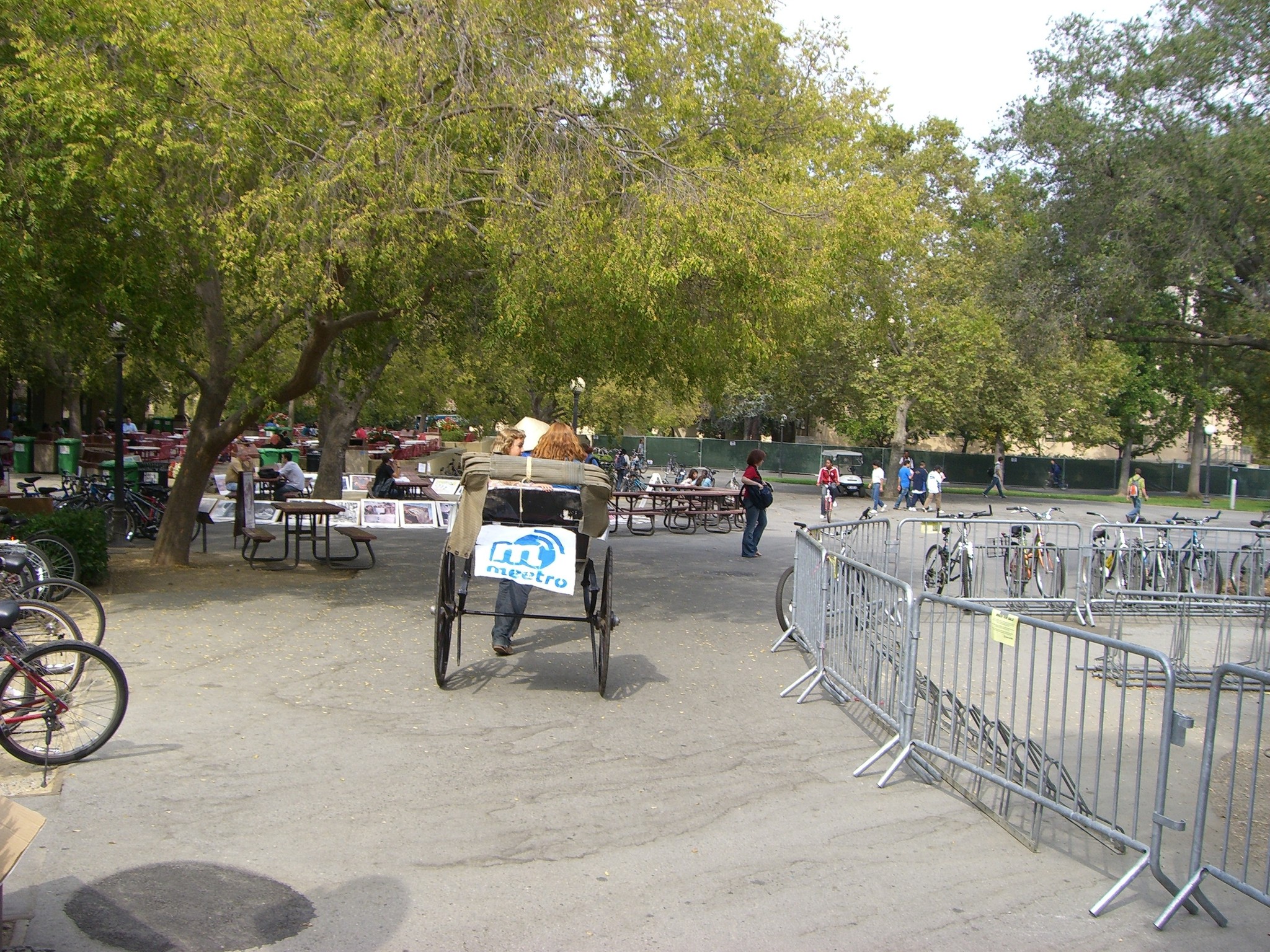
[{"xmin": 987, "ymin": 538, "xmax": 1008, "ymax": 558}]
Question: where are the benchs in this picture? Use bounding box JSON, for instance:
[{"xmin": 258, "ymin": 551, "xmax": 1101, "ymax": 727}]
[
  {"xmin": 459, "ymin": 452, "xmax": 611, "ymax": 536},
  {"xmin": 366, "ymin": 485, "xmax": 376, "ymax": 499},
  {"xmin": 240, "ymin": 525, "xmax": 276, "ymax": 572},
  {"xmin": 607, "ymin": 499, "xmax": 748, "ymax": 536},
  {"xmin": 225, "ymin": 488, "xmax": 238, "ymax": 497},
  {"xmin": 422, "ymin": 487, "xmax": 448, "ymax": 501},
  {"xmin": 336, "ymin": 527, "xmax": 375, "ymax": 567},
  {"xmin": 283, "ymin": 477, "xmax": 313, "ymax": 498}
]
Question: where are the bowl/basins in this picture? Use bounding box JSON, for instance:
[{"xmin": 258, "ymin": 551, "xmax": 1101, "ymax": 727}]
[{"xmin": 400, "ymin": 474, "xmax": 404, "ymax": 476}]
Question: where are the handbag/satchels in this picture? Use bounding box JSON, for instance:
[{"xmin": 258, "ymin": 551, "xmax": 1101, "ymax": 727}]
[
  {"xmin": 258, "ymin": 468, "xmax": 280, "ymax": 479},
  {"xmin": 837, "ymin": 486, "xmax": 844, "ymax": 493}
]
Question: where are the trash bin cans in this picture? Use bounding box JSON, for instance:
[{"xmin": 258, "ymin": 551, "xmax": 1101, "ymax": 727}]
[
  {"xmin": 55, "ymin": 438, "xmax": 81, "ymax": 477},
  {"xmin": 10, "ymin": 436, "xmax": 37, "ymax": 473},
  {"xmin": 279, "ymin": 448, "xmax": 300, "ymax": 464},
  {"xmin": 278, "ymin": 427, "xmax": 292, "ymax": 440},
  {"xmin": 98, "ymin": 460, "xmax": 139, "ymax": 493},
  {"xmin": 264, "ymin": 426, "xmax": 277, "ymax": 437},
  {"xmin": 137, "ymin": 462, "xmax": 171, "ymax": 498},
  {"xmin": 163, "ymin": 417, "xmax": 173, "ymax": 433},
  {"xmin": 151, "ymin": 416, "xmax": 163, "ymax": 433},
  {"xmin": 258, "ymin": 448, "xmax": 279, "ymax": 466}
]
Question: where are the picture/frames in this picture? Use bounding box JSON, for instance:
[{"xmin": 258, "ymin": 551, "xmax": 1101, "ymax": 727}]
[
  {"xmin": 349, "ymin": 474, "xmax": 376, "ymax": 492},
  {"xmin": 198, "ymin": 498, "xmax": 461, "ymax": 530},
  {"xmin": 342, "ymin": 476, "xmax": 349, "ymax": 492},
  {"xmin": 302, "ymin": 474, "xmax": 317, "ymax": 491}
]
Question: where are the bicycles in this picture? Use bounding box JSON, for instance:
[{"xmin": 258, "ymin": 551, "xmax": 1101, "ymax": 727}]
[
  {"xmin": 0, "ymin": 535, "xmax": 129, "ymax": 787},
  {"xmin": 1081, "ymin": 510, "xmax": 1224, "ymax": 600},
  {"xmin": 726, "ymin": 465, "xmax": 743, "ymax": 490},
  {"xmin": 675, "ymin": 463, "xmax": 691, "ymax": 483},
  {"xmin": 922, "ymin": 505, "xmax": 994, "ymax": 610},
  {"xmin": 1042, "ymin": 472, "xmax": 1068, "ymax": 491},
  {"xmin": 1230, "ymin": 519, "xmax": 1270, "ymax": 596},
  {"xmin": 704, "ymin": 465, "xmax": 720, "ymax": 487},
  {"xmin": 0, "ymin": 469, "xmax": 201, "ymax": 545},
  {"xmin": 665, "ymin": 454, "xmax": 679, "ymax": 474},
  {"xmin": 820, "ymin": 483, "xmax": 836, "ymax": 522},
  {"xmin": 593, "ymin": 447, "xmax": 667, "ymax": 505},
  {"xmin": 1003, "ymin": 505, "xmax": 1067, "ymax": 597},
  {"xmin": 776, "ymin": 505, "xmax": 876, "ymax": 641}
]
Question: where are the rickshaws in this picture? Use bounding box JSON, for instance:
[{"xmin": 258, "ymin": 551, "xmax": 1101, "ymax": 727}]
[{"xmin": 429, "ymin": 450, "xmax": 620, "ymax": 696}]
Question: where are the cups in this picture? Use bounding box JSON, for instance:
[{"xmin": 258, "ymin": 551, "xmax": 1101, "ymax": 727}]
[{"xmin": 254, "ymin": 467, "xmax": 260, "ymax": 477}]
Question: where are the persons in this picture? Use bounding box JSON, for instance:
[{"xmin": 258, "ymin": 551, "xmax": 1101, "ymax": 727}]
[
  {"xmin": 490, "ymin": 424, "xmax": 644, "ymax": 655},
  {"xmin": 2, "ymin": 410, "xmax": 476, "ymax": 502},
  {"xmin": 893, "ymin": 451, "xmax": 946, "ymax": 513},
  {"xmin": 692, "ymin": 470, "xmax": 712, "ymax": 501},
  {"xmin": 680, "ymin": 469, "xmax": 698, "ymax": 500},
  {"xmin": 868, "ymin": 460, "xmax": 887, "ymax": 513},
  {"xmin": 1049, "ymin": 459, "xmax": 1061, "ymax": 484},
  {"xmin": 741, "ymin": 449, "xmax": 767, "ymax": 558},
  {"xmin": 983, "ymin": 457, "xmax": 1007, "ymax": 498},
  {"xmin": 817, "ymin": 459, "xmax": 840, "ymax": 519},
  {"xmin": 1126, "ymin": 468, "xmax": 1148, "ymax": 523}
]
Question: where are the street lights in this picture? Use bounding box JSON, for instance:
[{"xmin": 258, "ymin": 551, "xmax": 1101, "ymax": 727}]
[
  {"xmin": 698, "ymin": 434, "xmax": 703, "ymax": 466},
  {"xmin": 109, "ymin": 322, "xmax": 129, "ymax": 543},
  {"xmin": 569, "ymin": 376, "xmax": 586, "ymax": 434},
  {"xmin": 1202, "ymin": 424, "xmax": 1215, "ymax": 509},
  {"xmin": 777, "ymin": 414, "xmax": 788, "ymax": 480}
]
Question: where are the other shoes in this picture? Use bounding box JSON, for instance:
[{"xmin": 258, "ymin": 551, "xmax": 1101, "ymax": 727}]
[
  {"xmin": 870, "ymin": 509, "xmax": 877, "ymax": 513},
  {"xmin": 1126, "ymin": 515, "xmax": 1131, "ymax": 522},
  {"xmin": 982, "ymin": 493, "xmax": 988, "ymax": 498},
  {"xmin": 820, "ymin": 514, "xmax": 824, "ymax": 519},
  {"xmin": 492, "ymin": 645, "xmax": 513, "ymax": 656},
  {"xmin": 908, "ymin": 507, "xmax": 917, "ymax": 512},
  {"xmin": 832, "ymin": 500, "xmax": 837, "ymax": 507},
  {"xmin": 893, "ymin": 507, "xmax": 901, "ymax": 510},
  {"xmin": 921, "ymin": 507, "xmax": 928, "ymax": 513},
  {"xmin": 999, "ymin": 495, "xmax": 1007, "ymax": 498},
  {"xmin": 754, "ymin": 551, "xmax": 761, "ymax": 557},
  {"xmin": 936, "ymin": 509, "xmax": 944, "ymax": 513},
  {"xmin": 881, "ymin": 504, "xmax": 887, "ymax": 513}
]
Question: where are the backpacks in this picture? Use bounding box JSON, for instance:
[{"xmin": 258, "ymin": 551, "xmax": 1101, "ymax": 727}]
[
  {"xmin": 372, "ymin": 477, "xmax": 395, "ymax": 498},
  {"xmin": 1129, "ymin": 477, "xmax": 1141, "ymax": 498},
  {"xmin": 739, "ymin": 478, "xmax": 773, "ymax": 509},
  {"xmin": 986, "ymin": 464, "xmax": 1001, "ymax": 477}
]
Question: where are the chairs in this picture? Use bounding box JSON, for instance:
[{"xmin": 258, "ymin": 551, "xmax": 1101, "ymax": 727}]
[
  {"xmin": 123, "ymin": 430, "xmax": 239, "ymax": 461},
  {"xmin": 366, "ymin": 433, "xmax": 439, "ymax": 460}
]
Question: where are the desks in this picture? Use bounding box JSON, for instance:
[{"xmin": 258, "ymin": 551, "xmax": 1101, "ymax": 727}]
[
  {"xmin": 125, "ymin": 446, "xmax": 161, "ymax": 461},
  {"xmin": 417, "ymin": 435, "xmax": 439, "ymax": 441},
  {"xmin": 167, "ymin": 435, "xmax": 184, "ymax": 444},
  {"xmin": 270, "ymin": 501, "xmax": 346, "ymax": 569},
  {"xmin": 175, "ymin": 445, "xmax": 187, "ymax": 456},
  {"xmin": 385, "ymin": 444, "xmax": 413, "ymax": 448},
  {"xmin": 123, "ymin": 431, "xmax": 147, "ymax": 445},
  {"xmin": 367, "ymin": 450, "xmax": 386, "ymax": 460},
  {"xmin": 144, "ymin": 437, "xmax": 174, "ymax": 457},
  {"xmin": 376, "ymin": 446, "xmax": 406, "ymax": 451},
  {"xmin": 612, "ymin": 483, "xmax": 746, "ymax": 497},
  {"xmin": 244, "ymin": 436, "xmax": 271, "ymax": 446},
  {"xmin": 393, "ymin": 470, "xmax": 429, "ymax": 499},
  {"xmin": 404, "ymin": 440, "xmax": 426, "ymax": 445},
  {"xmin": 251, "ymin": 474, "xmax": 280, "ymax": 500}
]
